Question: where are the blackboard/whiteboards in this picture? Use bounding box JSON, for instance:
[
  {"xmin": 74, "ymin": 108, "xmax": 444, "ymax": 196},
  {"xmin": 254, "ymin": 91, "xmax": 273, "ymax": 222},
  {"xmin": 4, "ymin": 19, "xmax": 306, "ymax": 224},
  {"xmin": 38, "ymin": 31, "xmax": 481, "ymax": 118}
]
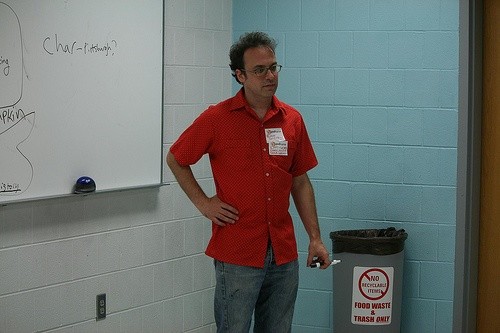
[{"xmin": 1, "ymin": 0, "xmax": 164, "ymax": 205}]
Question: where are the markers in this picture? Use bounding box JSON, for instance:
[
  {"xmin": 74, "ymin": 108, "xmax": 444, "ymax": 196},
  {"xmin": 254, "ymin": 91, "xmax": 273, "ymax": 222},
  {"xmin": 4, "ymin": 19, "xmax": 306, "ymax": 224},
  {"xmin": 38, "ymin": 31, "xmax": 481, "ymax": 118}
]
[{"xmin": 310, "ymin": 259, "xmax": 342, "ymax": 268}]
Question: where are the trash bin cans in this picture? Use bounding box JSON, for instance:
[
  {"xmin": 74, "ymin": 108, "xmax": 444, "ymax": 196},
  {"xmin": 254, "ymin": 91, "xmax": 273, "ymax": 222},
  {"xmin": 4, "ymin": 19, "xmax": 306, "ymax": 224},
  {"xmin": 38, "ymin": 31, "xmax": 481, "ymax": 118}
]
[{"xmin": 330, "ymin": 229, "xmax": 404, "ymax": 333}]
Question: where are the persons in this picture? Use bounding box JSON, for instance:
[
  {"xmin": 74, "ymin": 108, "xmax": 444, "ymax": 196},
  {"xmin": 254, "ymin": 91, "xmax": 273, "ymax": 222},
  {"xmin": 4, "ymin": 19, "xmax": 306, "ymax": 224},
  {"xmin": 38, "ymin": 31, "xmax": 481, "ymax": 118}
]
[{"xmin": 166, "ymin": 32, "xmax": 331, "ymax": 333}]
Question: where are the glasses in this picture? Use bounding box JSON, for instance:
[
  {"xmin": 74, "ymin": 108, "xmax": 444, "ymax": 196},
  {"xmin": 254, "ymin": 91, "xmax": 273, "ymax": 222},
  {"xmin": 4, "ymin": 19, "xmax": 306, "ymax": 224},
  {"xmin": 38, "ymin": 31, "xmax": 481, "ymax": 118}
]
[{"xmin": 240, "ymin": 64, "xmax": 283, "ymax": 74}]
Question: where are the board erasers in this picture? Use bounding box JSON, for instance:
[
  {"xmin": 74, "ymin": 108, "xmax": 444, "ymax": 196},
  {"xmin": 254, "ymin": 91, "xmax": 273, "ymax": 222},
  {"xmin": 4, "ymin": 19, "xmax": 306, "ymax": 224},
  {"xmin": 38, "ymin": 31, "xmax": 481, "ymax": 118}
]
[{"xmin": 75, "ymin": 177, "xmax": 96, "ymax": 194}]
